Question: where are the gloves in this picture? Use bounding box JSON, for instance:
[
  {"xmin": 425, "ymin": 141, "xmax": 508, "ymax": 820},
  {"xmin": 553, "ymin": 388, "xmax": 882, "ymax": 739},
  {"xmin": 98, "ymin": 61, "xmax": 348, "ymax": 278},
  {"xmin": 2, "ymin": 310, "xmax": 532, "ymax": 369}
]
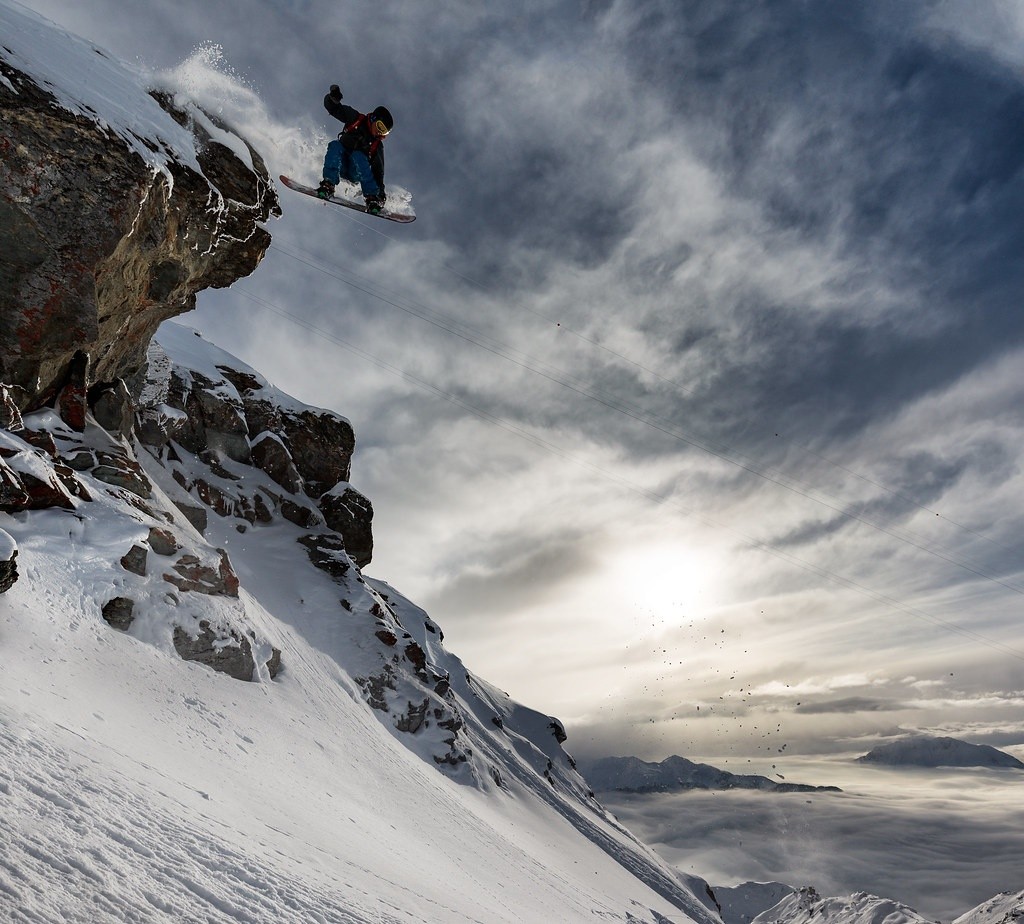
[
  {"xmin": 378, "ymin": 185, "xmax": 387, "ymax": 203},
  {"xmin": 329, "ymin": 84, "xmax": 344, "ymax": 104}
]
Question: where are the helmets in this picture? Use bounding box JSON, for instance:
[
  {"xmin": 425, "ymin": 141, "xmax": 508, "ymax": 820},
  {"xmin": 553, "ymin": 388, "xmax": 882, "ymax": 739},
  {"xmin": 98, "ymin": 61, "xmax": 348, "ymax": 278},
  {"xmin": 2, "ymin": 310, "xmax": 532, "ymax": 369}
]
[{"xmin": 369, "ymin": 106, "xmax": 394, "ymax": 131}]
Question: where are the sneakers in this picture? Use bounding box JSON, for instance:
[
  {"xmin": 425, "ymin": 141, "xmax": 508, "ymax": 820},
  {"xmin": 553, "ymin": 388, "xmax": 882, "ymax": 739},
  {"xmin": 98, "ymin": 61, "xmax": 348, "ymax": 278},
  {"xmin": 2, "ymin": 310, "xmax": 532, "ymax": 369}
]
[
  {"xmin": 366, "ymin": 196, "xmax": 384, "ymax": 213},
  {"xmin": 317, "ymin": 180, "xmax": 336, "ymax": 198}
]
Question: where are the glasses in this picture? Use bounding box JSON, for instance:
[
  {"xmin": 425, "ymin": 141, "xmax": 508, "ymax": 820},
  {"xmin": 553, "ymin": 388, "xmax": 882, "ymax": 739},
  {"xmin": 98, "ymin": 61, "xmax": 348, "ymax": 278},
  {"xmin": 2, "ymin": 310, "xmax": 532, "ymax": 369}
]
[{"xmin": 376, "ymin": 120, "xmax": 390, "ymax": 138}]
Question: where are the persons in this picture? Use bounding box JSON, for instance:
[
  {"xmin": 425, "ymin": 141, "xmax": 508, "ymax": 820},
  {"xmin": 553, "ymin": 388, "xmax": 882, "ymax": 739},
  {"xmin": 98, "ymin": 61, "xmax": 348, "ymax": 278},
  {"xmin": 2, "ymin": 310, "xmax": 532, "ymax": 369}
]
[{"xmin": 318, "ymin": 85, "xmax": 393, "ymax": 213}]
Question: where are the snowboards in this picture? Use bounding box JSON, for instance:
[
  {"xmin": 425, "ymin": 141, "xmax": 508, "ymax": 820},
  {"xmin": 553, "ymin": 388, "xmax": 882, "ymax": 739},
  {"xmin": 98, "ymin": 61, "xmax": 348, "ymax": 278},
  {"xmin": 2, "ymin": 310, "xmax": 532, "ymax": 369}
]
[{"xmin": 279, "ymin": 174, "xmax": 417, "ymax": 223}]
